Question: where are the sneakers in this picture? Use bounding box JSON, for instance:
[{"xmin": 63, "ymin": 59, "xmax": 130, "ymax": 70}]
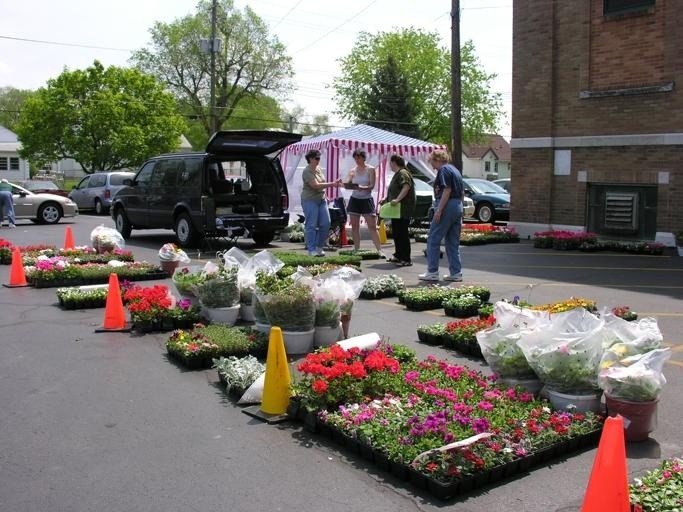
[
  {"xmin": 440, "ymin": 271, "xmax": 465, "ymax": 283},
  {"xmin": 308, "ymin": 247, "xmax": 326, "ymax": 257},
  {"xmin": 8, "ymin": 223, "xmax": 16, "ymax": 228},
  {"xmin": 416, "ymin": 270, "xmax": 442, "ymax": 282}
]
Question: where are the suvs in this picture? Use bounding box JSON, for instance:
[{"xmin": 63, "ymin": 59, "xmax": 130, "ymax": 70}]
[{"xmin": 109, "ymin": 128, "xmax": 305, "ymax": 248}]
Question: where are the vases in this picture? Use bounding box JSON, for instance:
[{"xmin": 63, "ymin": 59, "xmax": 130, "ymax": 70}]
[
  {"xmin": 602, "ymin": 389, "xmax": 661, "ymax": 442},
  {"xmin": 544, "ymin": 387, "xmax": 604, "ymax": 414},
  {"xmin": 161, "ymin": 261, "xmax": 180, "ymax": 278}
]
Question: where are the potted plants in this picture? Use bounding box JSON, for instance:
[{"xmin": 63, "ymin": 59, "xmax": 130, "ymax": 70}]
[
  {"xmin": 174, "ymin": 248, "xmax": 362, "ymax": 354},
  {"xmin": 359, "ymin": 273, "xmax": 406, "ymax": 299},
  {"xmin": 212, "ymin": 354, "xmax": 267, "ymax": 396},
  {"xmin": 337, "ymin": 248, "xmax": 380, "ymax": 260}
]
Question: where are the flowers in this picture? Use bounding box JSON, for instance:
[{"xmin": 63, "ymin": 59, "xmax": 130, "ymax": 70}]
[
  {"xmin": 484, "ymin": 298, "xmax": 667, "ymax": 400},
  {"xmin": 0, "ymin": 227, "xmax": 169, "ymax": 289},
  {"xmin": 629, "ymin": 457, "xmax": 683, "ymax": 511},
  {"xmin": 582, "ymin": 238, "xmax": 665, "ymax": 255},
  {"xmin": 123, "ymin": 283, "xmax": 207, "ymax": 335},
  {"xmin": 165, "ymin": 323, "xmax": 269, "ymax": 372},
  {"xmin": 397, "ymin": 283, "xmax": 495, "ymax": 319},
  {"xmin": 158, "ymin": 244, "xmax": 186, "ymax": 260},
  {"xmin": 56, "ymin": 281, "xmax": 133, "ymax": 311},
  {"xmin": 410, "ymin": 222, "xmax": 521, "ymax": 245},
  {"xmin": 417, "ymin": 316, "xmax": 496, "ymax": 359},
  {"xmin": 293, "ymin": 340, "xmax": 605, "ymax": 502},
  {"xmin": 532, "ymin": 229, "xmax": 596, "ymax": 251}
]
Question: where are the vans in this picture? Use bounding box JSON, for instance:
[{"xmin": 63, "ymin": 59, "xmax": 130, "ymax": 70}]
[{"xmin": 68, "ymin": 170, "xmax": 137, "ymax": 214}]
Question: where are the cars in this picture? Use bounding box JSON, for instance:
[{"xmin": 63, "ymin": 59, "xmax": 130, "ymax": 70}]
[
  {"xmin": 0, "ymin": 179, "xmax": 78, "ymax": 223},
  {"xmin": 410, "ymin": 175, "xmax": 513, "ymax": 226}
]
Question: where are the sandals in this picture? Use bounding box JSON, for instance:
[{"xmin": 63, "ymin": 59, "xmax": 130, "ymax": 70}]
[{"xmin": 386, "ymin": 255, "xmax": 413, "ymax": 268}]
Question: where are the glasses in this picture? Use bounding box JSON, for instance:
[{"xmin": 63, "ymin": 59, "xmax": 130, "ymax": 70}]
[{"xmin": 310, "ymin": 156, "xmax": 322, "ymax": 161}]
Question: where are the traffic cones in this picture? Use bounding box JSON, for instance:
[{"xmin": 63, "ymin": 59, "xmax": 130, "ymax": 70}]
[
  {"xmin": 575, "ymin": 416, "xmax": 634, "ymax": 511},
  {"xmin": 2, "ymin": 246, "xmax": 31, "ymax": 289},
  {"xmin": 94, "ymin": 272, "xmax": 135, "ymax": 334},
  {"xmin": 242, "ymin": 324, "xmax": 299, "ymax": 424},
  {"xmin": 337, "ymin": 223, "xmax": 349, "ymax": 245},
  {"xmin": 378, "ymin": 220, "xmax": 393, "ymax": 245},
  {"xmin": 61, "ymin": 226, "xmax": 77, "ymax": 251}
]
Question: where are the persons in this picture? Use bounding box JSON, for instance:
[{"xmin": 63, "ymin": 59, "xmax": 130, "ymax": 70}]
[
  {"xmin": 0, "ymin": 178, "xmax": 15, "ymax": 228},
  {"xmin": 417, "ymin": 147, "xmax": 465, "ymax": 283},
  {"xmin": 343, "ymin": 147, "xmax": 383, "ymax": 254},
  {"xmin": 300, "ymin": 148, "xmax": 341, "ymax": 256},
  {"xmin": 375, "ymin": 154, "xmax": 416, "ymax": 268}
]
